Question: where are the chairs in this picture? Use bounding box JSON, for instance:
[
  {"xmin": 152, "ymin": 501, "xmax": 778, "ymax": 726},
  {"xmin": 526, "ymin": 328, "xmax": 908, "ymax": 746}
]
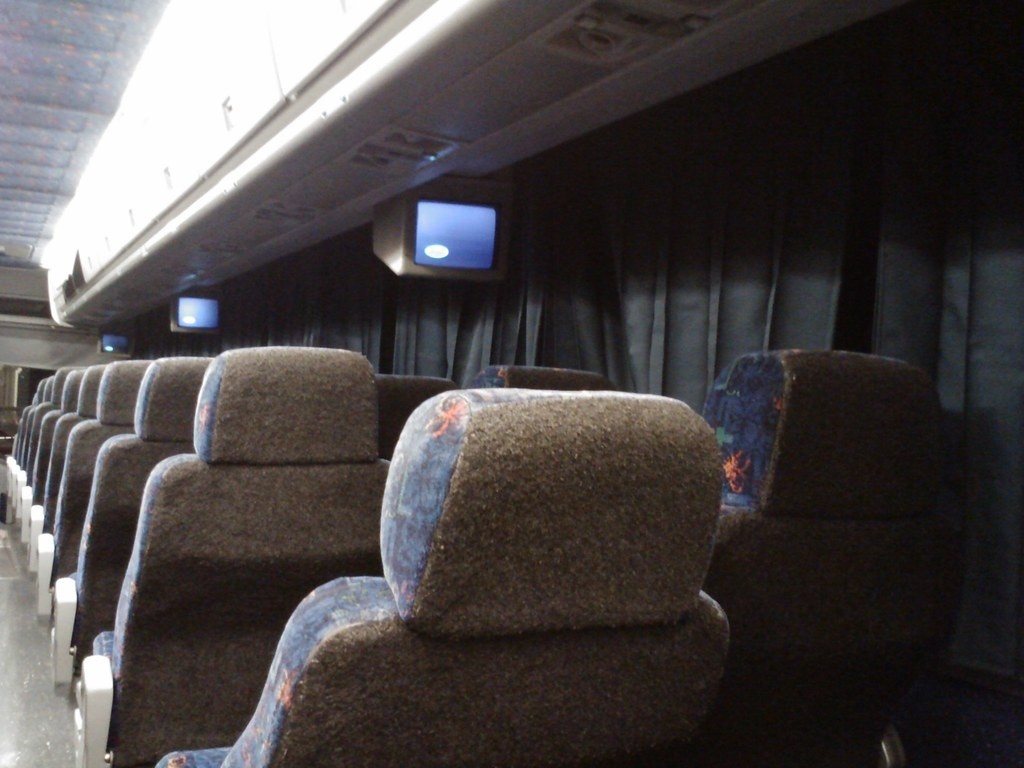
[{"xmin": 6, "ymin": 346, "xmax": 963, "ymax": 768}]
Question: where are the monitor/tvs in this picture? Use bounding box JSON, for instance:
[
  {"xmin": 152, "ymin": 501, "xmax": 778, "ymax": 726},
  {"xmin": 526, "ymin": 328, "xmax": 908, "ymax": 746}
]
[
  {"xmin": 169, "ymin": 290, "xmax": 224, "ymax": 334},
  {"xmin": 372, "ymin": 174, "xmax": 514, "ymax": 279},
  {"xmin": 97, "ymin": 327, "xmax": 135, "ymax": 358}
]
